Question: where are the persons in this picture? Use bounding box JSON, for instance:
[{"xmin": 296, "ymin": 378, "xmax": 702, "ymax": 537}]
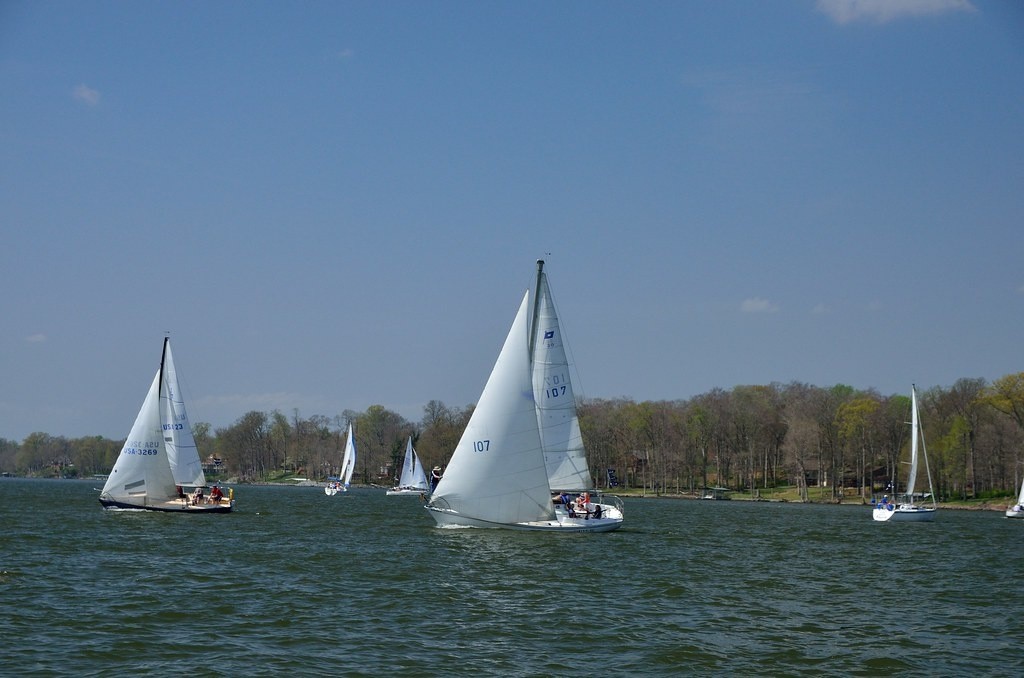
[
  {"xmin": 429, "ymin": 464, "xmax": 442, "ymax": 494},
  {"xmin": 177, "ymin": 484, "xmax": 224, "ymax": 505},
  {"xmin": 567, "ymin": 491, "xmax": 602, "ymax": 520},
  {"xmin": 328, "ymin": 481, "xmax": 348, "ymax": 491}
]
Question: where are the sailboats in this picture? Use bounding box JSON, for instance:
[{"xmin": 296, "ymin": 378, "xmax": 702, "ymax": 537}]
[
  {"xmin": 1005, "ymin": 475, "xmax": 1024, "ymax": 518},
  {"xmin": 420, "ymin": 258, "xmax": 626, "ymax": 533},
  {"xmin": 873, "ymin": 383, "xmax": 939, "ymax": 522},
  {"xmin": 98, "ymin": 330, "xmax": 236, "ymax": 514},
  {"xmin": 386, "ymin": 435, "xmax": 430, "ymax": 496},
  {"xmin": 324, "ymin": 421, "xmax": 357, "ymax": 495}
]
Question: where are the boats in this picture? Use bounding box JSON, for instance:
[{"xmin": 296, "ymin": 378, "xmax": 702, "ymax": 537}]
[{"xmin": 704, "ymin": 487, "xmax": 732, "ymax": 501}]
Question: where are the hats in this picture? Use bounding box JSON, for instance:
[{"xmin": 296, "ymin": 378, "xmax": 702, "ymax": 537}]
[
  {"xmin": 884, "ymin": 494, "xmax": 888, "ymax": 497},
  {"xmin": 433, "ymin": 466, "xmax": 441, "ymax": 471}
]
